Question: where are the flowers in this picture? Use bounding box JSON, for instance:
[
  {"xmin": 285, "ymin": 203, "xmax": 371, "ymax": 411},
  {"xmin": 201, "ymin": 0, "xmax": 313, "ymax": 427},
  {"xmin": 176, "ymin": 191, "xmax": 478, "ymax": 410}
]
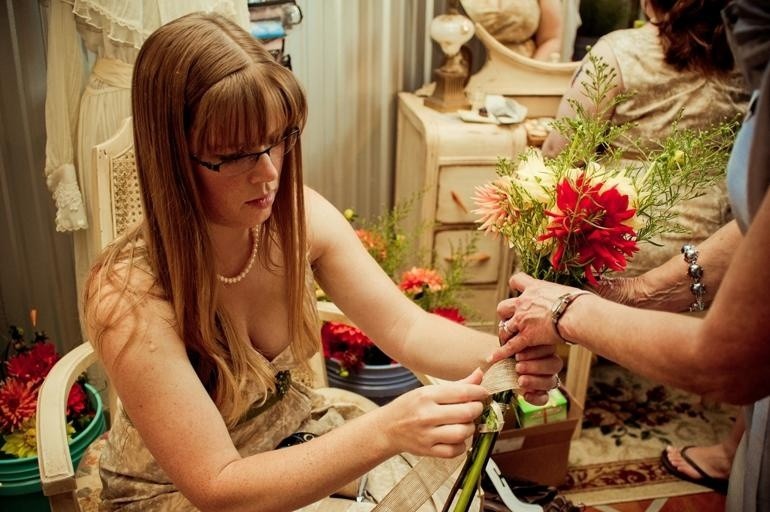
[{"xmin": 0, "ymin": 322, "xmax": 96, "ymax": 460}]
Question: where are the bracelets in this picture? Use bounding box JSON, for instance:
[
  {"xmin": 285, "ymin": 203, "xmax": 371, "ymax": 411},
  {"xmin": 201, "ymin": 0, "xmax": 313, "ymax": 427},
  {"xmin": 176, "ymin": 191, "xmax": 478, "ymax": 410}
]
[{"xmin": 677, "ymin": 240, "xmax": 711, "ymax": 314}]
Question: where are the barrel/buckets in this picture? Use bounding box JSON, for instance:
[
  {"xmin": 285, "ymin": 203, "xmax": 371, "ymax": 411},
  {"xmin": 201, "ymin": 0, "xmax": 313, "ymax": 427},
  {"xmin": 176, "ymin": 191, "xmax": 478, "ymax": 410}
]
[{"xmin": 322, "ymin": 350, "xmax": 422, "ymax": 400}]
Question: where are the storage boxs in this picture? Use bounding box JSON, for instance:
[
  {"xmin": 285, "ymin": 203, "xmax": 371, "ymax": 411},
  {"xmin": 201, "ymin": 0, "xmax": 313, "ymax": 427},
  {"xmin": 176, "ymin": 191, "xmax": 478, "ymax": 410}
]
[{"xmin": 480, "ymin": 380, "xmax": 585, "ymax": 487}]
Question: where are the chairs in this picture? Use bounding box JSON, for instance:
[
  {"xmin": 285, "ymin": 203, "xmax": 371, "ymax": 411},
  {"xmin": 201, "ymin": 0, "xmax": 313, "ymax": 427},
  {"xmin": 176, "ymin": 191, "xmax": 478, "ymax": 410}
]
[{"xmin": 37, "ymin": 119, "xmax": 383, "ymax": 512}]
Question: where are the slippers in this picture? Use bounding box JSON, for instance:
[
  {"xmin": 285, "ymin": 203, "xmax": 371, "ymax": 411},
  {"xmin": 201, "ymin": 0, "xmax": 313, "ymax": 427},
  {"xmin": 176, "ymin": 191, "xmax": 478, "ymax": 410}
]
[{"xmin": 662, "ymin": 444, "xmax": 731, "ymax": 498}]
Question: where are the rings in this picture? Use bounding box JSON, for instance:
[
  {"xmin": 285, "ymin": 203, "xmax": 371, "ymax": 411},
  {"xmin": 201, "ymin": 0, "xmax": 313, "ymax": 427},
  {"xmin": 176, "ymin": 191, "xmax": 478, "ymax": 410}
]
[{"xmin": 497, "ymin": 318, "xmax": 514, "ymax": 336}]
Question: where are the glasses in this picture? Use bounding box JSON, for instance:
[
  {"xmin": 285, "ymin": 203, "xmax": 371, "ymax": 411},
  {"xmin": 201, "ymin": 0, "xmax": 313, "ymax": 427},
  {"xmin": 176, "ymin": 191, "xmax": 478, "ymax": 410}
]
[{"xmin": 192, "ymin": 125, "xmax": 301, "ymax": 179}]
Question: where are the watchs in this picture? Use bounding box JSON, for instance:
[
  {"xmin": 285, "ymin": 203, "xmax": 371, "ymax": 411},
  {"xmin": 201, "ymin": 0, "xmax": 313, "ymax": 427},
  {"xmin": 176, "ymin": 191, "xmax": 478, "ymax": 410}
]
[{"xmin": 549, "ymin": 288, "xmax": 593, "ymax": 345}]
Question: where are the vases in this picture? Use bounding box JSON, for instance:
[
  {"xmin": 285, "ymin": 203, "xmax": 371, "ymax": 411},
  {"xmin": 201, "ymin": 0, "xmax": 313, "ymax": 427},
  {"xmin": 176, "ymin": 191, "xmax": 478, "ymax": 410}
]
[{"xmin": 325, "ymin": 360, "xmax": 421, "ymax": 406}]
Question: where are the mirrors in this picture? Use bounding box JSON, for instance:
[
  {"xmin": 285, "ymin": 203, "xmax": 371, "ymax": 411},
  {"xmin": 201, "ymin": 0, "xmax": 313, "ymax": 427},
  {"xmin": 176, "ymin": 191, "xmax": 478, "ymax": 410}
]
[{"xmin": 457, "ymin": 1, "xmax": 648, "ymax": 74}]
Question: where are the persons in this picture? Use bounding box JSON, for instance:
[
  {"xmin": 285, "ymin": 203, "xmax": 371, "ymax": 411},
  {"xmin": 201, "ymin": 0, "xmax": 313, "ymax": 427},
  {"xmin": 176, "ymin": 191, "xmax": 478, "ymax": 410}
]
[
  {"xmin": 43, "ymin": 0, "xmax": 254, "ymax": 356},
  {"xmin": 480, "ymin": 2, "xmax": 769, "ymax": 509},
  {"xmin": 656, "ymin": 407, "xmax": 747, "ymax": 497},
  {"xmin": 67, "ymin": 9, "xmax": 567, "ymax": 512},
  {"xmin": 467, "ymin": 0, "xmax": 586, "ymax": 65},
  {"xmin": 543, "ymin": 0, "xmax": 756, "ymax": 171}
]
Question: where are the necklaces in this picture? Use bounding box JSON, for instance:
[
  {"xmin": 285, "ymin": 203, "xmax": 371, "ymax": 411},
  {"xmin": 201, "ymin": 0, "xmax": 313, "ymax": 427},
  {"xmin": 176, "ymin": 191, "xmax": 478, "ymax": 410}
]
[{"xmin": 207, "ymin": 227, "xmax": 264, "ymax": 291}]
